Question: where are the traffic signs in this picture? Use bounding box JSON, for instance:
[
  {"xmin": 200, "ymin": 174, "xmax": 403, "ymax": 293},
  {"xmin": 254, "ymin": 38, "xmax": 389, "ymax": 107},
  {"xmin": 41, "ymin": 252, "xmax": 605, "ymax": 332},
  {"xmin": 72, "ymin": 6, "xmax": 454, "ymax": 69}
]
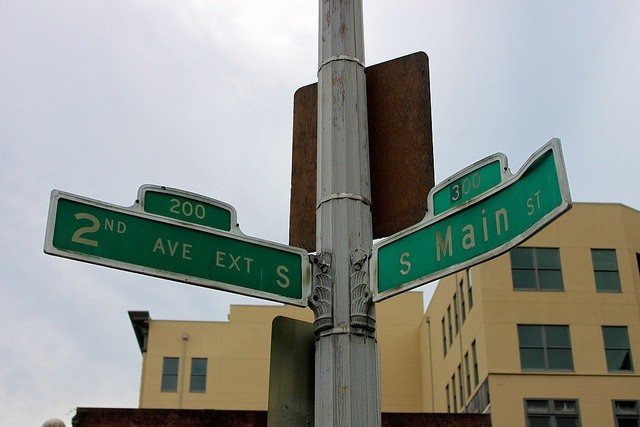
[
  {"xmin": 42, "ymin": 182, "xmax": 312, "ymax": 307},
  {"xmin": 374, "ymin": 135, "xmax": 571, "ymax": 303}
]
